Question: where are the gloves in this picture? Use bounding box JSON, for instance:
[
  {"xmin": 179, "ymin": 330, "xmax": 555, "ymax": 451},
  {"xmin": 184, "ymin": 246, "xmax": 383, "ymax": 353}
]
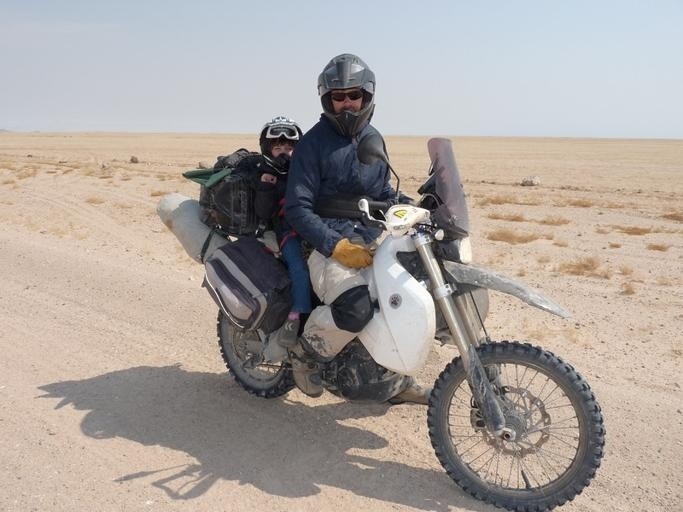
[{"xmin": 332, "ymin": 238, "xmax": 373, "ymax": 269}]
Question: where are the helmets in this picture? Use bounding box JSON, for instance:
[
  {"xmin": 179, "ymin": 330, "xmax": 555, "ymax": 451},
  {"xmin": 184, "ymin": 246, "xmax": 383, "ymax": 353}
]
[
  {"xmin": 260, "ymin": 117, "xmax": 303, "ymax": 172},
  {"xmin": 318, "ymin": 55, "xmax": 375, "ymax": 137}
]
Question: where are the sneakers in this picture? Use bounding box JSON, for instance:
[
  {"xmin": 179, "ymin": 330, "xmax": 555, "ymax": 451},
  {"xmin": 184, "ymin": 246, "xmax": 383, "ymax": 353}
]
[
  {"xmin": 278, "ymin": 320, "xmax": 301, "ymax": 348},
  {"xmin": 287, "ymin": 343, "xmax": 323, "ymax": 397},
  {"xmin": 389, "ymin": 383, "xmax": 432, "ymax": 405}
]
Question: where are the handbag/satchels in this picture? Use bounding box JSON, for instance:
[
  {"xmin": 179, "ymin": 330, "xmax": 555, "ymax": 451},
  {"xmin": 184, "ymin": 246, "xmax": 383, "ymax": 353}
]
[
  {"xmin": 199, "ymin": 149, "xmax": 261, "ymax": 235},
  {"xmin": 202, "ymin": 238, "xmax": 292, "ymax": 334}
]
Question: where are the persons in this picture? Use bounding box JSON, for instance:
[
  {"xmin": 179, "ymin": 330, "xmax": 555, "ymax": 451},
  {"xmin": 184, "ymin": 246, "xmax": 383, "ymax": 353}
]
[
  {"xmin": 253, "ymin": 115, "xmax": 314, "ymax": 348},
  {"xmin": 286, "ymin": 54, "xmax": 434, "ymax": 406}
]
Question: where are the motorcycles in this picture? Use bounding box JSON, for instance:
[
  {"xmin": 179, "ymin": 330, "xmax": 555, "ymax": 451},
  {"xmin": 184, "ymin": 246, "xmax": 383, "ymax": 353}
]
[{"xmin": 199, "ymin": 132, "xmax": 610, "ymax": 509}]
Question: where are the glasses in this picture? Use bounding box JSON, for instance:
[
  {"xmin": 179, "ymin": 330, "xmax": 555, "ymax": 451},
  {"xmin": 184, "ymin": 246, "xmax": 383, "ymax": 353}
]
[
  {"xmin": 331, "ymin": 91, "xmax": 363, "ymax": 101},
  {"xmin": 266, "ymin": 126, "xmax": 300, "ymax": 141}
]
